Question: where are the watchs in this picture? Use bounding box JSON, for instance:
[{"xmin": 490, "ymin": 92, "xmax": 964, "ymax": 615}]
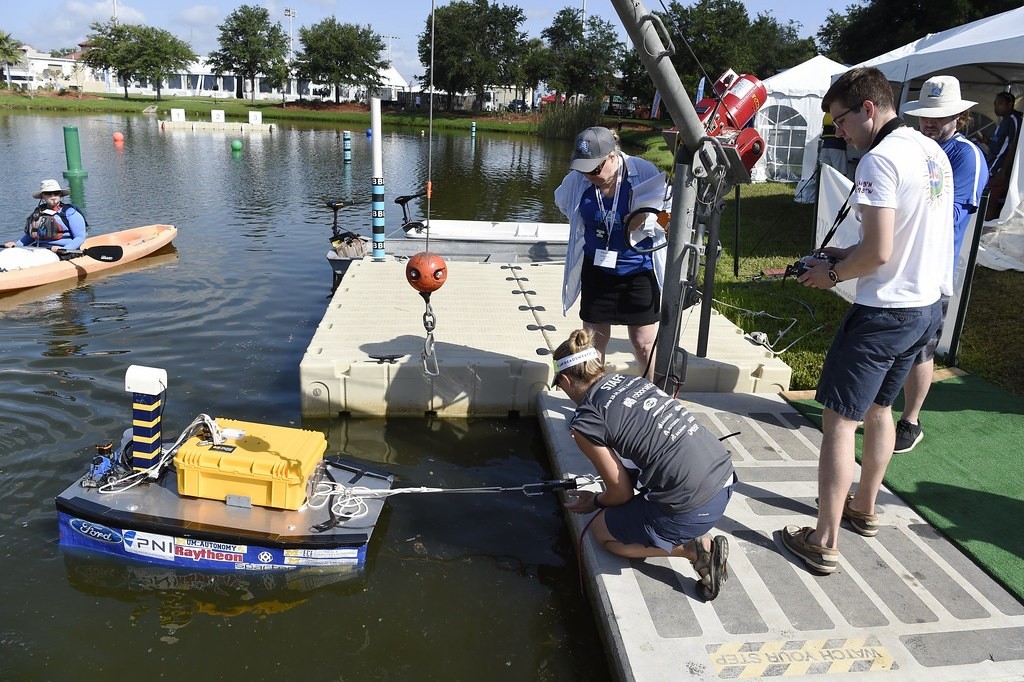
[{"xmin": 828, "ymin": 264, "xmax": 844, "ymax": 283}]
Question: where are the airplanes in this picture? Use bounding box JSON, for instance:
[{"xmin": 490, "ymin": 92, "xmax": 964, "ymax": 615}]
[{"xmin": 1, "ymin": 242, "xmax": 181, "ymax": 314}]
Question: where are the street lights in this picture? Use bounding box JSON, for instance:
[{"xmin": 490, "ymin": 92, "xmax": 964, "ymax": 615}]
[{"xmin": 283, "ymin": 8, "xmax": 297, "ymax": 62}]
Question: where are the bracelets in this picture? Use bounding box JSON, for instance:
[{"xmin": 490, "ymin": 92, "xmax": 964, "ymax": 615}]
[{"xmin": 594, "ymin": 493, "xmax": 608, "ymax": 509}]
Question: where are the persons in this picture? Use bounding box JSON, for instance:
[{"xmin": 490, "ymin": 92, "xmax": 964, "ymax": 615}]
[
  {"xmin": 554, "ymin": 127, "xmax": 673, "ymax": 384},
  {"xmin": 820, "ymin": 112, "xmax": 848, "ymax": 177},
  {"xmin": 355, "ymin": 90, "xmax": 365, "ymax": 103},
  {"xmin": 985, "ymin": 92, "xmax": 1022, "ymax": 221},
  {"xmin": 415, "ymin": 94, "xmax": 421, "ymax": 111},
  {"xmin": 860, "ymin": 75, "xmax": 989, "ymax": 454},
  {"xmin": 782, "ymin": 67, "xmax": 954, "ymax": 573},
  {"xmin": 5, "ymin": 179, "xmax": 86, "ymax": 262},
  {"xmin": 551, "ymin": 327, "xmax": 739, "ymax": 601}
]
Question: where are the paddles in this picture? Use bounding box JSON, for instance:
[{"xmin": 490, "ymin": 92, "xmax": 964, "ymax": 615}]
[{"xmin": 0, "ymin": 244, "xmax": 124, "ymax": 262}]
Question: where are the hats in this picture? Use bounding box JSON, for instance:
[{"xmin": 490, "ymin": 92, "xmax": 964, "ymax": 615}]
[
  {"xmin": 550, "ymin": 348, "xmax": 599, "ymax": 388},
  {"xmin": 33, "ymin": 180, "xmax": 69, "ymax": 198},
  {"xmin": 901, "ymin": 76, "xmax": 979, "ymax": 119},
  {"xmin": 570, "ymin": 126, "xmax": 616, "ymax": 173}
]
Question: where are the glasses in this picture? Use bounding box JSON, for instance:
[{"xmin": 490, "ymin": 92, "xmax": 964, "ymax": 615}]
[
  {"xmin": 586, "ymin": 159, "xmax": 607, "ymax": 176},
  {"xmin": 42, "ymin": 191, "xmax": 61, "ymax": 197},
  {"xmin": 832, "ymin": 101, "xmax": 865, "ymax": 129}
]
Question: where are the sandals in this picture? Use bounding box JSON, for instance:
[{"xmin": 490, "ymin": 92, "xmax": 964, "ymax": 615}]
[{"xmin": 691, "ymin": 534, "xmax": 729, "ymax": 602}]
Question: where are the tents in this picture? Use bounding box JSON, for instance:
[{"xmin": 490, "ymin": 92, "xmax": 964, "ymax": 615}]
[
  {"xmin": 750, "ymin": 55, "xmax": 849, "ymax": 184},
  {"xmin": 831, "ymin": 5, "xmax": 1024, "ymax": 274}
]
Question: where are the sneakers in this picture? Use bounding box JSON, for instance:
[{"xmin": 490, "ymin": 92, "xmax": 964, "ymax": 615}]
[
  {"xmin": 782, "ymin": 526, "xmax": 838, "ymax": 574},
  {"xmin": 894, "ymin": 416, "xmax": 924, "ymax": 453},
  {"xmin": 816, "ymin": 492, "xmax": 879, "ymax": 535}
]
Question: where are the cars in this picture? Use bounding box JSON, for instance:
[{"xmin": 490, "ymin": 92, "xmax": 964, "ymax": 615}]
[{"xmin": 509, "ymin": 99, "xmax": 529, "ymax": 113}]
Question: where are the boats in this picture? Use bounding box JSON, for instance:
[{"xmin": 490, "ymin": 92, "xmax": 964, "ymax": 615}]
[
  {"xmin": 53, "ymin": 363, "xmax": 399, "ymax": 576},
  {"xmin": 326, "ymin": 196, "xmax": 568, "ymax": 276},
  {"xmin": 1, "ymin": 223, "xmax": 179, "ymax": 291},
  {"xmin": 393, "ymin": 187, "xmax": 571, "ymax": 243}
]
